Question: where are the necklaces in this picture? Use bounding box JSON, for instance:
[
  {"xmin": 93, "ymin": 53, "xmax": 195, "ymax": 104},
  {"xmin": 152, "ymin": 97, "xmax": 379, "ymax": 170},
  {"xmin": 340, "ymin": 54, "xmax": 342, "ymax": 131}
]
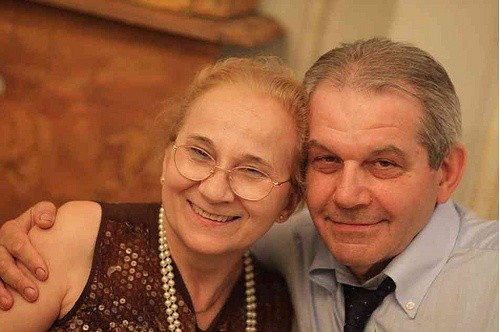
[{"xmin": 158, "ymin": 205, "xmax": 257, "ymax": 332}]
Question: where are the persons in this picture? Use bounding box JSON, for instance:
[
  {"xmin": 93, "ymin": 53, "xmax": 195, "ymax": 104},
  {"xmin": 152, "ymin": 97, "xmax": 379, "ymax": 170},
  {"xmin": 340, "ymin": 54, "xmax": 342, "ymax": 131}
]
[{"xmin": 0, "ymin": 38, "xmax": 499, "ymax": 332}]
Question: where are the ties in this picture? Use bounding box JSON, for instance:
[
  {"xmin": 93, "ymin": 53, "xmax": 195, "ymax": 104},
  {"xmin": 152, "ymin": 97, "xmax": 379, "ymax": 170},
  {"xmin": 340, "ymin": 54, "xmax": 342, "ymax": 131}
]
[{"xmin": 341, "ymin": 276, "xmax": 396, "ymax": 332}]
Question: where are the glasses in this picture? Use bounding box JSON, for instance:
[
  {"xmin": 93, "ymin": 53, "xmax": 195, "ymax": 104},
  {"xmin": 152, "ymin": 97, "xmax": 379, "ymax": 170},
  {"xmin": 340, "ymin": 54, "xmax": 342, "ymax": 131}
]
[{"xmin": 171, "ymin": 140, "xmax": 292, "ymax": 202}]
